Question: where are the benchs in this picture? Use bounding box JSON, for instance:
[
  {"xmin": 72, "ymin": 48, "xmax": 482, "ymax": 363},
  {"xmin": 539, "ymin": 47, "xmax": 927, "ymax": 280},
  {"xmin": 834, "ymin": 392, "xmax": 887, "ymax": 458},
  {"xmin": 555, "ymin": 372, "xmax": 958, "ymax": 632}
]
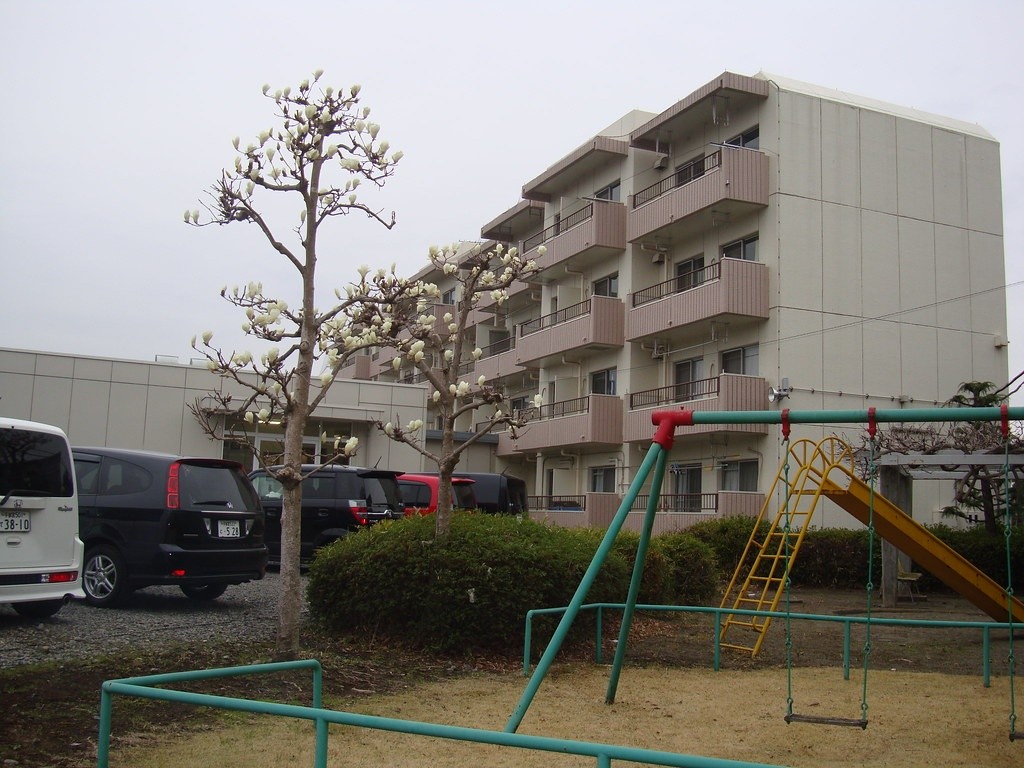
[{"xmin": 896, "ymin": 558, "xmax": 922, "ymax": 606}]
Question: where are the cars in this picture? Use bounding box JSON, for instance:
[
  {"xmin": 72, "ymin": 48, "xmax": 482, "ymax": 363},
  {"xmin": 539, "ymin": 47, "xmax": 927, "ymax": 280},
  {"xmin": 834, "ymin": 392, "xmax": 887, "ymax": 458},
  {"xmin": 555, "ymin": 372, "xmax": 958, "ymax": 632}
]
[{"xmin": 548, "ymin": 500, "xmax": 584, "ymax": 512}]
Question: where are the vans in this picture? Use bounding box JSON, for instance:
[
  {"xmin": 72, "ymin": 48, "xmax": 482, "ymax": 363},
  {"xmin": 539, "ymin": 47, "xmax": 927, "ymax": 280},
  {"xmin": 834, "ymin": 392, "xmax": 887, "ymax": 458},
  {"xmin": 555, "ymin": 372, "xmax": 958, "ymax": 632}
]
[{"xmin": 1, "ymin": 418, "xmax": 86, "ymax": 621}]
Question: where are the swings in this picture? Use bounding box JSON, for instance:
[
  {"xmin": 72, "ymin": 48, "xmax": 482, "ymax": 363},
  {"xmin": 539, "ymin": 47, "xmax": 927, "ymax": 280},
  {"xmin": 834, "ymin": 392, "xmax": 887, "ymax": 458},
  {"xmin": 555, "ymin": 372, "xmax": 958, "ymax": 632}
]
[
  {"xmin": 779, "ymin": 405, "xmax": 877, "ymax": 730},
  {"xmin": 1000, "ymin": 404, "xmax": 1024, "ymax": 742}
]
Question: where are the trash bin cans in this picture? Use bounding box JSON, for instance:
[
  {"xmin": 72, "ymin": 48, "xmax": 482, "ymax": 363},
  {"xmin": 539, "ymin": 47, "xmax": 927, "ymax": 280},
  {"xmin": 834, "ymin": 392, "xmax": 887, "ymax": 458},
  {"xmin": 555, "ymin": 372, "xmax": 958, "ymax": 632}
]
[{"xmin": 547, "ymin": 501, "xmax": 582, "ymax": 510}]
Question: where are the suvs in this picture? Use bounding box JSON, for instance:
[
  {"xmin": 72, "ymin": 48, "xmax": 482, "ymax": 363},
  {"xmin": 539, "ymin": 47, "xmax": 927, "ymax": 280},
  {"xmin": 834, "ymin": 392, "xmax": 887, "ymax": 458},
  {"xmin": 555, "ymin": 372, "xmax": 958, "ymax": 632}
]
[
  {"xmin": 29, "ymin": 445, "xmax": 271, "ymax": 607},
  {"xmin": 394, "ymin": 477, "xmax": 476, "ymax": 522},
  {"xmin": 242, "ymin": 464, "xmax": 405, "ymax": 566},
  {"xmin": 418, "ymin": 472, "xmax": 531, "ymax": 519}
]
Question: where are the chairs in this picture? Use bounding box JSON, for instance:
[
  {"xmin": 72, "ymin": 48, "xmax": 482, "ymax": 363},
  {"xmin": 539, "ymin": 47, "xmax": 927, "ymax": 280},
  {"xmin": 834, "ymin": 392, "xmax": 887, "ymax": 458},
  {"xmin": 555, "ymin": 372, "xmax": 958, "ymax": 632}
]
[
  {"xmin": 303, "ymin": 478, "xmax": 318, "ymax": 497},
  {"xmin": 316, "ymin": 479, "xmax": 328, "ymax": 497}
]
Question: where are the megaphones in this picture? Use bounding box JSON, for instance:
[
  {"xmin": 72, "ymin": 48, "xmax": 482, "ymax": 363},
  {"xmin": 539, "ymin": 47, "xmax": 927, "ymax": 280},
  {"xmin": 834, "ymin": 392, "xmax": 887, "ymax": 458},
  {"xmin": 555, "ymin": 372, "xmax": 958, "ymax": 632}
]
[{"xmin": 767, "ymin": 386, "xmax": 790, "ymax": 402}]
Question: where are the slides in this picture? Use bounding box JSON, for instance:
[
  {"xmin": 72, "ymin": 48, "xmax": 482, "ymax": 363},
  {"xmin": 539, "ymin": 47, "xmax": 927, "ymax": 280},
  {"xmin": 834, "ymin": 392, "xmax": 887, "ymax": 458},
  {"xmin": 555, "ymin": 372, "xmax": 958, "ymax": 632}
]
[{"xmin": 821, "ymin": 475, "xmax": 1024, "ymax": 623}]
